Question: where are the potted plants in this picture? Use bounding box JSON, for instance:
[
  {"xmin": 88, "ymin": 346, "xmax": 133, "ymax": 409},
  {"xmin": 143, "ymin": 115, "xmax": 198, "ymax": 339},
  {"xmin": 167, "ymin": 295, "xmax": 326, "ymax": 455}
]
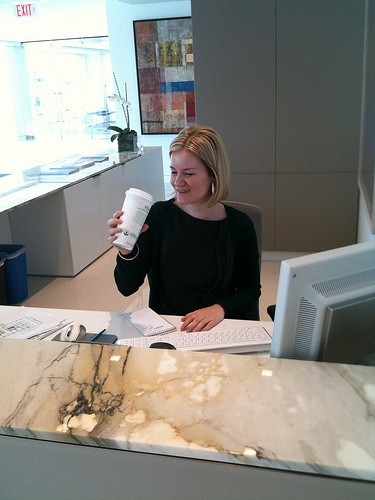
[{"xmin": 106, "ymin": 72, "xmax": 138, "ymax": 152}]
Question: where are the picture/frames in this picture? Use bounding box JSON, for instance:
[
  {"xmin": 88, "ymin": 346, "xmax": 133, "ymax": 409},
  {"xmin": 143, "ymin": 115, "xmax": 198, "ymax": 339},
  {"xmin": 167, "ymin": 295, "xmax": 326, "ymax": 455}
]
[{"xmin": 132, "ymin": 16, "xmax": 196, "ymax": 135}]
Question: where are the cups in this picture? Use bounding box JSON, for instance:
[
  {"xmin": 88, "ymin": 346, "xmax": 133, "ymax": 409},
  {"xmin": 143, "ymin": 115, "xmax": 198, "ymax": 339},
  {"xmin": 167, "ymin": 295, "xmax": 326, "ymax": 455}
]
[{"xmin": 112, "ymin": 187, "xmax": 154, "ymax": 252}]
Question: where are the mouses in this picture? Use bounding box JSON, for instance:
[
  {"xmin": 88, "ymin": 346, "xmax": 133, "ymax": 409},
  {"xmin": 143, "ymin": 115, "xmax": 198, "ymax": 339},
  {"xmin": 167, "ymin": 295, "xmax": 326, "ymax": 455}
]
[{"xmin": 60, "ymin": 322, "xmax": 87, "ymax": 342}]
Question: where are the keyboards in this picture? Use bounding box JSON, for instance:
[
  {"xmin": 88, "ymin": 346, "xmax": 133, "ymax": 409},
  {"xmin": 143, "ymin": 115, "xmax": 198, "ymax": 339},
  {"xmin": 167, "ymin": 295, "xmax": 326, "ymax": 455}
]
[{"xmin": 114, "ymin": 325, "xmax": 273, "ymax": 354}]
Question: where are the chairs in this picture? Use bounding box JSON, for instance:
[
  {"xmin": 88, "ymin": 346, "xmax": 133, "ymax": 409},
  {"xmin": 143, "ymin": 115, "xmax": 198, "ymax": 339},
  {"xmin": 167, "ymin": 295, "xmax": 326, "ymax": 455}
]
[{"xmin": 220, "ymin": 200, "xmax": 262, "ymax": 300}]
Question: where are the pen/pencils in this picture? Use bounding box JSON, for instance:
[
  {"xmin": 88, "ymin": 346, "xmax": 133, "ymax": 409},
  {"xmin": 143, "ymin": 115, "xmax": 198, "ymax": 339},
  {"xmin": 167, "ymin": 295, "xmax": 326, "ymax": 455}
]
[{"xmin": 90, "ymin": 328, "xmax": 106, "ymax": 342}]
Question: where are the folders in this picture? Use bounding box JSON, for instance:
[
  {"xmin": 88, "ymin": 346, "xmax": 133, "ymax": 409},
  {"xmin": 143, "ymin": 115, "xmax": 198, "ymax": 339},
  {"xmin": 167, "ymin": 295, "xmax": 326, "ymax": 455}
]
[{"xmin": 76, "ymin": 156, "xmax": 109, "ymax": 163}]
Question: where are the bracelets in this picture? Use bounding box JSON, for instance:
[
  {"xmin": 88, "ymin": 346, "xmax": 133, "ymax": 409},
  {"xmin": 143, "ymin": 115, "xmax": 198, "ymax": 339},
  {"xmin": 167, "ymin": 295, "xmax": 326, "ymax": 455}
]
[{"xmin": 117, "ymin": 242, "xmax": 139, "ymax": 261}]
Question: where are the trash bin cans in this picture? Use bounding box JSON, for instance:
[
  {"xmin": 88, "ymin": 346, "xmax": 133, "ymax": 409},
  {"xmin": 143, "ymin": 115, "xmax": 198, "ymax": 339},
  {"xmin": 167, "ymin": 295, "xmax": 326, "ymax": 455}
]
[
  {"xmin": 0, "ymin": 243, "xmax": 29, "ymax": 305},
  {"xmin": 0, "ymin": 255, "xmax": 10, "ymax": 306}
]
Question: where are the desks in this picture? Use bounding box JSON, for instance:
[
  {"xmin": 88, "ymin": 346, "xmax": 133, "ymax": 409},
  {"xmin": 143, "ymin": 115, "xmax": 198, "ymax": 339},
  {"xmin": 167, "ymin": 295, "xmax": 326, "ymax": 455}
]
[{"xmin": 0, "ymin": 304, "xmax": 273, "ymax": 357}]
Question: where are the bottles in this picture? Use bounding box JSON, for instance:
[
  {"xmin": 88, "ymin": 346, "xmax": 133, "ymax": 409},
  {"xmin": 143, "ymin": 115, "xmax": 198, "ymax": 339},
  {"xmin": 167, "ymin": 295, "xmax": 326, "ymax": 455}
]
[{"xmin": 14, "ymin": 134, "xmax": 41, "ymax": 184}]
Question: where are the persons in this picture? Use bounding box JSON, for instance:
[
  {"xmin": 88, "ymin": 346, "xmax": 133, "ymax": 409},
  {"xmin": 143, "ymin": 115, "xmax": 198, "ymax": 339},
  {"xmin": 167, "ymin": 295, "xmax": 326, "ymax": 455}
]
[{"xmin": 107, "ymin": 126, "xmax": 261, "ymax": 332}]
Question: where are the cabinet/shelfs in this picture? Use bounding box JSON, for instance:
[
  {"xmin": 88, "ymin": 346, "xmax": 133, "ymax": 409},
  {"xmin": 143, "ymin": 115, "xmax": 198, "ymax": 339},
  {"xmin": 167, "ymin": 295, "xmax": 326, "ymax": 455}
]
[{"xmin": 0, "ymin": 146, "xmax": 165, "ymax": 278}]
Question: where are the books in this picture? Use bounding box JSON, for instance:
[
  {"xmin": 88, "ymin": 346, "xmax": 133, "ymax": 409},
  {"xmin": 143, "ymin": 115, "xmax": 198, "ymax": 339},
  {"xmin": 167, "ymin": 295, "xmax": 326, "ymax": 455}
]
[{"xmin": 120, "ymin": 307, "xmax": 177, "ymax": 337}]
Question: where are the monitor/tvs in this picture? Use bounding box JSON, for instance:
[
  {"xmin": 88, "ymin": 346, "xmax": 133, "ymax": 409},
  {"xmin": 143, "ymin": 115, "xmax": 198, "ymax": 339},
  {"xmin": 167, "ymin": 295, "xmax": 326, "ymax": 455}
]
[{"xmin": 269, "ymin": 241, "xmax": 375, "ymax": 367}]
